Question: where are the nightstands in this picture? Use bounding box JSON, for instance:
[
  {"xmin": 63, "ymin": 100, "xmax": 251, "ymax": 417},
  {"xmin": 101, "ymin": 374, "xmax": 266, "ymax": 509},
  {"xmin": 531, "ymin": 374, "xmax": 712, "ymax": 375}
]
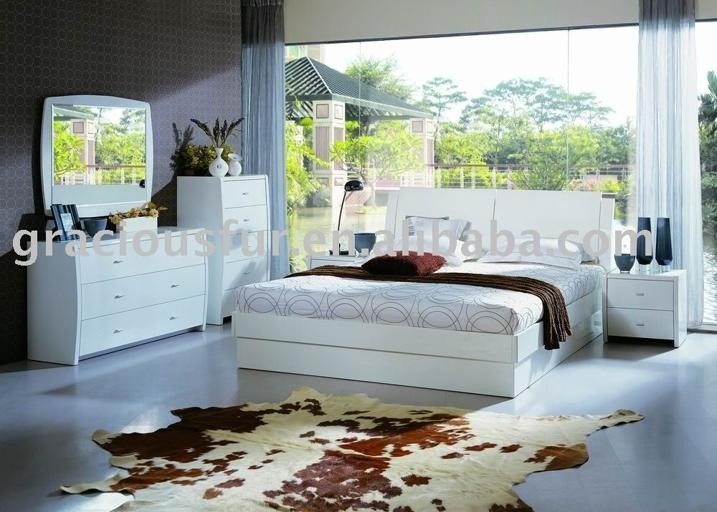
[
  {"xmin": 307, "ymin": 253, "xmax": 366, "ymax": 270},
  {"xmin": 601, "ymin": 264, "xmax": 690, "ymax": 347}
]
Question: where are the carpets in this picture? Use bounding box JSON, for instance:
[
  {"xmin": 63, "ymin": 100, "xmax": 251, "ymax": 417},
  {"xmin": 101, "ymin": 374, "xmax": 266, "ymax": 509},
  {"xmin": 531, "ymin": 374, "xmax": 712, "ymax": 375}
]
[{"xmin": 56, "ymin": 385, "xmax": 647, "ymax": 511}]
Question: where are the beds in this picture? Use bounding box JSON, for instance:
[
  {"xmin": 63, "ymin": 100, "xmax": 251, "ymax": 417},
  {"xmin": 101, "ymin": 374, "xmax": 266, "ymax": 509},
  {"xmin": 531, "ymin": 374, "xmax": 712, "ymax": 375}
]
[{"xmin": 231, "ymin": 184, "xmax": 615, "ymax": 400}]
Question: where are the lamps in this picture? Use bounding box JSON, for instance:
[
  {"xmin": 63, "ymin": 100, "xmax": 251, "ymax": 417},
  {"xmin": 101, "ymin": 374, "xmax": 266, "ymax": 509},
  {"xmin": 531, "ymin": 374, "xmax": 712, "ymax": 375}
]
[{"xmin": 327, "ymin": 179, "xmax": 364, "ymax": 256}]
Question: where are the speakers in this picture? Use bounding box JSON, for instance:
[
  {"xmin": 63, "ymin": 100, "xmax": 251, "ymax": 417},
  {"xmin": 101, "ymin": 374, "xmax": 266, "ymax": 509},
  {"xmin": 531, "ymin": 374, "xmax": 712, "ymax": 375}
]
[
  {"xmin": 656, "ymin": 217, "xmax": 672, "ymax": 274},
  {"xmin": 637, "ymin": 216, "xmax": 653, "ymax": 271}
]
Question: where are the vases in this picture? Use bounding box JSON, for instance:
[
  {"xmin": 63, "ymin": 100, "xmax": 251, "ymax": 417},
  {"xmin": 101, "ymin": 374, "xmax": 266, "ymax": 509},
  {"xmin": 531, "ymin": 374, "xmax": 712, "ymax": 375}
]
[
  {"xmin": 169, "ymin": 115, "xmax": 248, "ymax": 176},
  {"xmin": 636, "ymin": 216, "xmax": 673, "ymax": 276},
  {"xmin": 116, "ymin": 217, "xmax": 158, "ymax": 232}
]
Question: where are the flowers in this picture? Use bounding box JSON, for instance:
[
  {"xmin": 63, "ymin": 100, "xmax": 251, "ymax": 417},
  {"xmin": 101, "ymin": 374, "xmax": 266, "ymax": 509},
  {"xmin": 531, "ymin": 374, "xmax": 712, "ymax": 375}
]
[{"xmin": 108, "ymin": 201, "xmax": 168, "ymax": 224}]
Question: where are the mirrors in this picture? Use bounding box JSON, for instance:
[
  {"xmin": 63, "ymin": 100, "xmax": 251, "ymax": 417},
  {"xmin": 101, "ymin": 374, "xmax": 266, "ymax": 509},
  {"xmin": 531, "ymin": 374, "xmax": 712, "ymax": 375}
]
[{"xmin": 38, "ymin": 94, "xmax": 155, "ymax": 218}]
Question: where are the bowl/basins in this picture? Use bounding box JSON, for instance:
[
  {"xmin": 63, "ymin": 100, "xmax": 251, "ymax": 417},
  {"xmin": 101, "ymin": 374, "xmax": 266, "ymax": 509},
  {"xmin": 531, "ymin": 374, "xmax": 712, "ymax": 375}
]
[{"xmin": 84, "ymin": 218, "xmax": 107, "ymax": 239}]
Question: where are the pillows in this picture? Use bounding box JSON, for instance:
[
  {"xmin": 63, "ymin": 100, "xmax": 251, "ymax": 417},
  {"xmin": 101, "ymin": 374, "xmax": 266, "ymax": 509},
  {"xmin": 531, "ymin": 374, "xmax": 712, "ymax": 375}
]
[{"xmin": 361, "ymin": 216, "xmax": 584, "ymax": 276}]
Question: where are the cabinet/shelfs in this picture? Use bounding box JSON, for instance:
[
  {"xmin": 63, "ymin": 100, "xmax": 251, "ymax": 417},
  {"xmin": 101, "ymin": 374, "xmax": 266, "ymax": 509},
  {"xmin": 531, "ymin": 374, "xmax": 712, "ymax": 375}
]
[
  {"xmin": 174, "ymin": 171, "xmax": 273, "ymax": 326},
  {"xmin": 22, "ymin": 227, "xmax": 208, "ymax": 366}
]
